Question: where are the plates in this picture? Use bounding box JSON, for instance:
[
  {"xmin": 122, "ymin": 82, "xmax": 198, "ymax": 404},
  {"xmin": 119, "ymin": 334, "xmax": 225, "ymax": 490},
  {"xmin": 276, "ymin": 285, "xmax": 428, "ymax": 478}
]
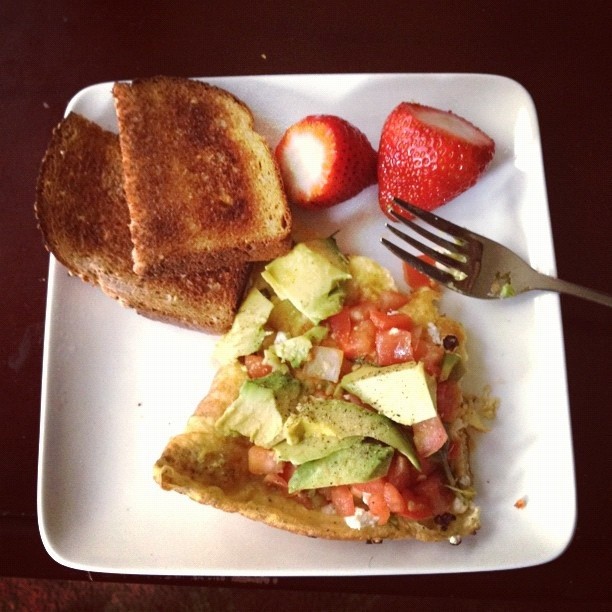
[{"xmin": 40, "ymin": 73, "xmax": 578, "ymax": 578}]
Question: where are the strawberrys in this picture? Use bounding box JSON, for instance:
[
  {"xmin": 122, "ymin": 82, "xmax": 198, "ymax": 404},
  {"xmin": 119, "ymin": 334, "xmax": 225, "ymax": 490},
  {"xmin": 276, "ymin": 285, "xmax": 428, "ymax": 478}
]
[
  {"xmin": 279, "ymin": 112, "xmax": 375, "ymax": 207},
  {"xmin": 379, "ymin": 101, "xmax": 494, "ymax": 220}
]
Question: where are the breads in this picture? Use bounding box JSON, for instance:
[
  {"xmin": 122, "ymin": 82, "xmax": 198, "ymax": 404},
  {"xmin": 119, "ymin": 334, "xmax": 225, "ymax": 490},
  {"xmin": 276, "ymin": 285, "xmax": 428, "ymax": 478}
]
[
  {"xmin": 108, "ymin": 72, "xmax": 297, "ymax": 274},
  {"xmin": 28, "ymin": 109, "xmax": 233, "ymax": 334}
]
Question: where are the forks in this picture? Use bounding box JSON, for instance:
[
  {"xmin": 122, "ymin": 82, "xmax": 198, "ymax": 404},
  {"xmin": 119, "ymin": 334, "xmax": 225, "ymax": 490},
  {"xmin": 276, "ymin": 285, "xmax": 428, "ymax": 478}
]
[{"xmin": 378, "ymin": 192, "xmax": 610, "ymax": 310}]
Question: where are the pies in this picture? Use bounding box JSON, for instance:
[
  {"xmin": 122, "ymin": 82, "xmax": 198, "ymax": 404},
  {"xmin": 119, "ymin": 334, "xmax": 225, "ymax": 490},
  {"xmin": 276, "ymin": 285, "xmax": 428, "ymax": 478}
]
[{"xmin": 153, "ymin": 234, "xmax": 504, "ymax": 550}]
